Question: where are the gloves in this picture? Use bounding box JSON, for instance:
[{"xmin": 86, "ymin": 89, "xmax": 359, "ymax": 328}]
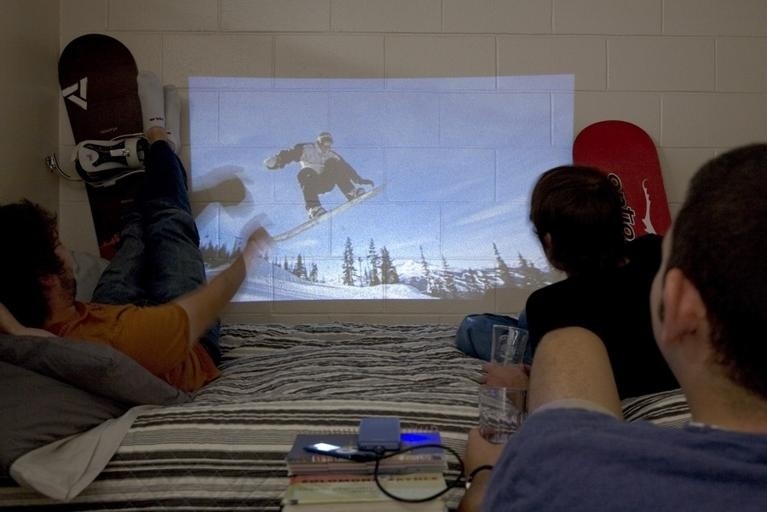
[
  {"xmin": 264, "ymin": 154, "xmax": 286, "ymax": 169},
  {"xmin": 359, "ymin": 178, "xmax": 373, "ymax": 185}
]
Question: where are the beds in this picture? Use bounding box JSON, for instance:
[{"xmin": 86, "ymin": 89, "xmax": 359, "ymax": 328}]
[{"xmin": 0, "ymin": 323, "xmax": 701, "ymax": 512}]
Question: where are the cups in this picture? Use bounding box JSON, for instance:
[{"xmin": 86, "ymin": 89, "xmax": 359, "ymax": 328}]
[
  {"xmin": 477, "ymin": 385, "xmax": 526, "ymax": 447},
  {"xmin": 490, "ymin": 323, "xmax": 530, "ymax": 370}
]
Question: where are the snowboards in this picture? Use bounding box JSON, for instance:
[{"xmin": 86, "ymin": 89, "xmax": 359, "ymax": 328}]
[
  {"xmin": 57, "ymin": 33, "xmax": 148, "ymax": 262},
  {"xmin": 571, "ymin": 119, "xmax": 672, "ymax": 238},
  {"xmin": 273, "ymin": 184, "xmax": 380, "ymax": 241}
]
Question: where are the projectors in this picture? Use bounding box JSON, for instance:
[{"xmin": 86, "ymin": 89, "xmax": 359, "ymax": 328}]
[{"xmin": 357, "ymin": 416, "xmax": 401, "ymax": 451}]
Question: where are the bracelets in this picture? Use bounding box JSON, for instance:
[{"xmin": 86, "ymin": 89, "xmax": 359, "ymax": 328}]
[{"xmin": 465, "ymin": 465, "xmax": 496, "ymax": 490}]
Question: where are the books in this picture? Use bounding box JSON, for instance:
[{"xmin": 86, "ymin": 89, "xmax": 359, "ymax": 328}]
[{"xmin": 278, "ymin": 432, "xmax": 448, "ymax": 512}]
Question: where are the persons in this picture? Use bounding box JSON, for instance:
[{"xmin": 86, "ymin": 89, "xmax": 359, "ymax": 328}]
[
  {"xmin": 1, "ymin": 69, "xmax": 274, "ymax": 391},
  {"xmin": 455, "ymin": 162, "xmax": 681, "ymax": 404},
  {"xmin": 262, "ymin": 132, "xmax": 375, "ymax": 219},
  {"xmin": 453, "ymin": 140, "xmax": 765, "ymax": 511}
]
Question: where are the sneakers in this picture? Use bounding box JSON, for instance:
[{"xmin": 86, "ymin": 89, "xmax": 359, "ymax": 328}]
[
  {"xmin": 308, "ymin": 205, "xmax": 327, "ymax": 219},
  {"xmin": 345, "ymin": 188, "xmax": 365, "ymax": 201}
]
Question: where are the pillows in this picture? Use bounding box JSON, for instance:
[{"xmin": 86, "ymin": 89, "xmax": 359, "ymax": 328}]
[{"xmin": 1, "ymin": 335, "xmax": 191, "ymax": 474}]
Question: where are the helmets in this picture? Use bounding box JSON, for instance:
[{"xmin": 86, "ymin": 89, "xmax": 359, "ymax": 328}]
[{"xmin": 317, "ymin": 132, "xmax": 333, "ymax": 149}]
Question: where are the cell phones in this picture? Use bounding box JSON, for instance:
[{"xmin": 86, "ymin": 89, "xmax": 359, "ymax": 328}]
[{"xmin": 304, "ymin": 441, "xmax": 373, "ymax": 461}]
